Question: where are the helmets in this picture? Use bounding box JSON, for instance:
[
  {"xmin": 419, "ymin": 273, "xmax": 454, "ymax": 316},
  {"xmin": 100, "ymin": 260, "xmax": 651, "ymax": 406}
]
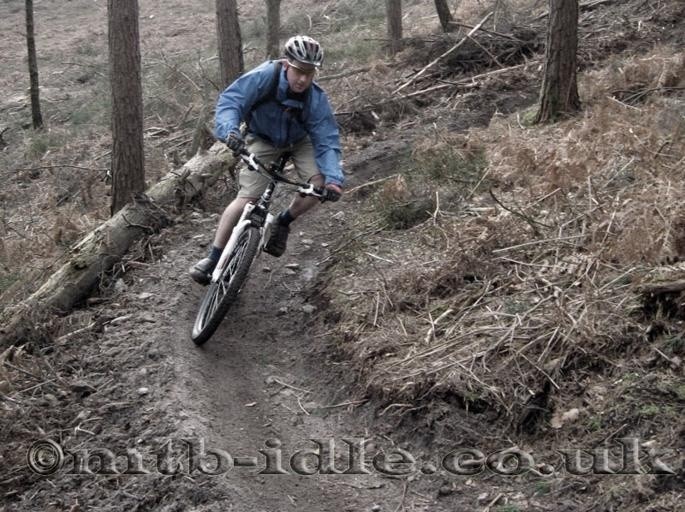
[{"xmin": 283, "ymin": 35, "xmax": 324, "ymax": 67}]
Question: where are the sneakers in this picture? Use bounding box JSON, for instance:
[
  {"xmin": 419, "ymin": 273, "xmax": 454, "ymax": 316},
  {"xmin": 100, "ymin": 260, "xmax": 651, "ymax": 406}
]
[
  {"xmin": 191, "ymin": 257, "xmax": 217, "ymax": 285},
  {"xmin": 263, "ymin": 212, "xmax": 289, "ymax": 257}
]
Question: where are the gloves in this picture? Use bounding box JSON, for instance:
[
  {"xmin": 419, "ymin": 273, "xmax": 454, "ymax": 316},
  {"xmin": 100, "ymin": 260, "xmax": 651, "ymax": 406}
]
[
  {"xmin": 318, "ymin": 183, "xmax": 343, "ymax": 204},
  {"xmin": 226, "ymin": 132, "xmax": 245, "ymax": 157}
]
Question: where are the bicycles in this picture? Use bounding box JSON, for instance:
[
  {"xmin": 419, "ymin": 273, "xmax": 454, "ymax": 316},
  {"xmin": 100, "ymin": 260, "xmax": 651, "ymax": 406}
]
[{"xmin": 190, "ymin": 135, "xmax": 342, "ymax": 348}]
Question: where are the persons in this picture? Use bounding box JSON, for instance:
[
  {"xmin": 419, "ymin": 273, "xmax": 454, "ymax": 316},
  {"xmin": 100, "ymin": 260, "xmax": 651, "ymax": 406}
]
[{"xmin": 190, "ymin": 36, "xmax": 348, "ymax": 285}]
